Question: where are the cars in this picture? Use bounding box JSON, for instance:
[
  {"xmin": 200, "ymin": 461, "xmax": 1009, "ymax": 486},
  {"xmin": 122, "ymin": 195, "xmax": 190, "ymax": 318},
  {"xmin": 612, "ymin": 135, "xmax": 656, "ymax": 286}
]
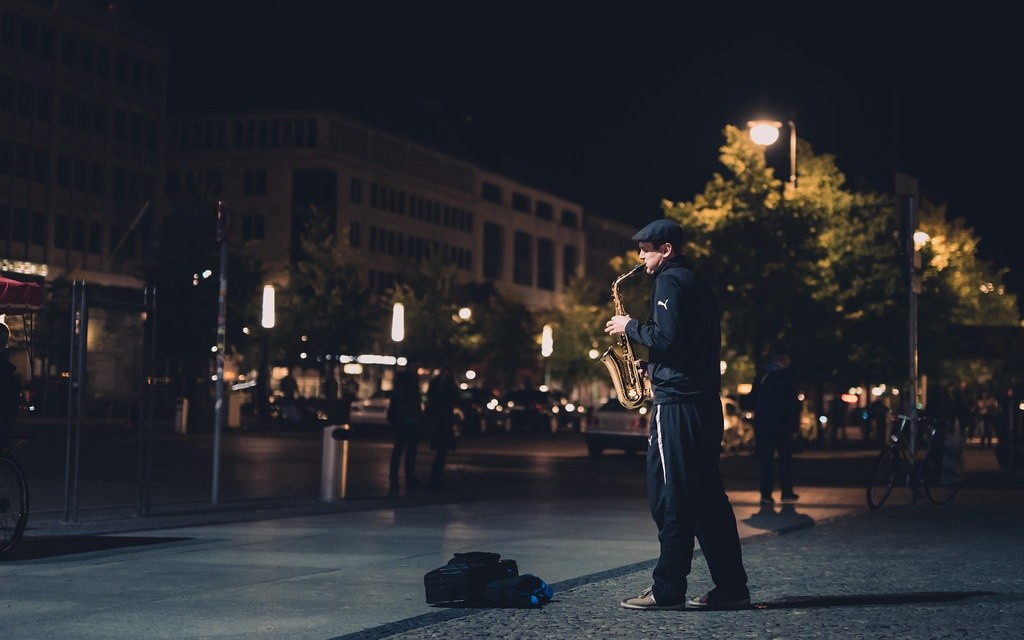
[
  {"xmin": 461, "ymin": 388, "xmax": 587, "ymax": 435},
  {"xmin": 348, "ymin": 390, "xmax": 464, "ymax": 439},
  {"xmin": 584, "ymin": 398, "xmax": 755, "ymax": 456},
  {"xmin": 800, "ymin": 399, "xmax": 831, "ymax": 448}
]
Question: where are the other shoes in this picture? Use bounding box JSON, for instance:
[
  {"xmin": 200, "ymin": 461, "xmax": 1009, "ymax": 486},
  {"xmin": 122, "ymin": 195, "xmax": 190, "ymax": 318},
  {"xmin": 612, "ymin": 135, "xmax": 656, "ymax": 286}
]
[
  {"xmin": 782, "ymin": 493, "xmax": 799, "ymax": 502},
  {"xmin": 760, "ymin": 497, "xmax": 774, "ymax": 503},
  {"xmin": 621, "ymin": 586, "xmax": 685, "ymax": 611},
  {"xmin": 688, "ymin": 585, "xmax": 751, "ymax": 609}
]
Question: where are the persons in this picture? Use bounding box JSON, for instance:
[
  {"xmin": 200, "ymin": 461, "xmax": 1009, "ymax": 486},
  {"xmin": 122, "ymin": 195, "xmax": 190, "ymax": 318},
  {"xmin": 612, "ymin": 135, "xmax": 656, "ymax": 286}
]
[
  {"xmin": 0, "ymin": 324, "xmax": 22, "ymax": 454},
  {"xmin": 387, "ymin": 371, "xmax": 422, "ymax": 495},
  {"xmin": 740, "ymin": 354, "xmax": 800, "ymax": 513},
  {"xmin": 827, "ymin": 381, "xmax": 1015, "ymax": 449},
  {"xmin": 423, "ymin": 367, "xmax": 465, "ymax": 474},
  {"xmin": 279, "ymin": 366, "xmax": 358, "ymax": 405},
  {"xmin": 605, "ymin": 218, "xmax": 751, "ymax": 610}
]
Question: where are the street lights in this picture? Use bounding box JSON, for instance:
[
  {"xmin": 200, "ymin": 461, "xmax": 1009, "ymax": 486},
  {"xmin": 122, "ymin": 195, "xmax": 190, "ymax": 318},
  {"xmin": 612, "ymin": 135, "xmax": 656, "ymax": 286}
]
[{"xmin": 746, "ymin": 110, "xmax": 797, "ymax": 186}]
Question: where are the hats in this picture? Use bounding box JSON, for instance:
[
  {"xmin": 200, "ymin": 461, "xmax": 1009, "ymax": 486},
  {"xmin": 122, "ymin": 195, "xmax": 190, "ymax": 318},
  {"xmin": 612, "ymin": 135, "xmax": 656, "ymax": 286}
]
[{"xmin": 631, "ymin": 219, "xmax": 685, "ymax": 243}]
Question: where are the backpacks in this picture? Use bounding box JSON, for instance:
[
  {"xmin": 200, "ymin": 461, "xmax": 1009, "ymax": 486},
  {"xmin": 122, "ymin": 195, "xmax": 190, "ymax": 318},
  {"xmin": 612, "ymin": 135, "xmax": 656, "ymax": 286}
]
[{"xmin": 424, "ymin": 552, "xmax": 554, "ymax": 608}]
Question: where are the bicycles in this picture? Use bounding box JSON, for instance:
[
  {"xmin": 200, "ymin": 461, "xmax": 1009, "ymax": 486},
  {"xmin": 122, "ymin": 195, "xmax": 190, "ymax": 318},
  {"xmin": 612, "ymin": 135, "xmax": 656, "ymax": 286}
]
[
  {"xmin": 865, "ymin": 414, "xmax": 964, "ymax": 509},
  {"xmin": 1, "ymin": 447, "xmax": 30, "ymax": 561}
]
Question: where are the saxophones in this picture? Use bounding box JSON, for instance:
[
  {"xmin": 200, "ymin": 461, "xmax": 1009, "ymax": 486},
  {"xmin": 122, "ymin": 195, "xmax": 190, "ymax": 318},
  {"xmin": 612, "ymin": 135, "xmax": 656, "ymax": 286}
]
[{"xmin": 594, "ymin": 260, "xmax": 649, "ymax": 411}]
